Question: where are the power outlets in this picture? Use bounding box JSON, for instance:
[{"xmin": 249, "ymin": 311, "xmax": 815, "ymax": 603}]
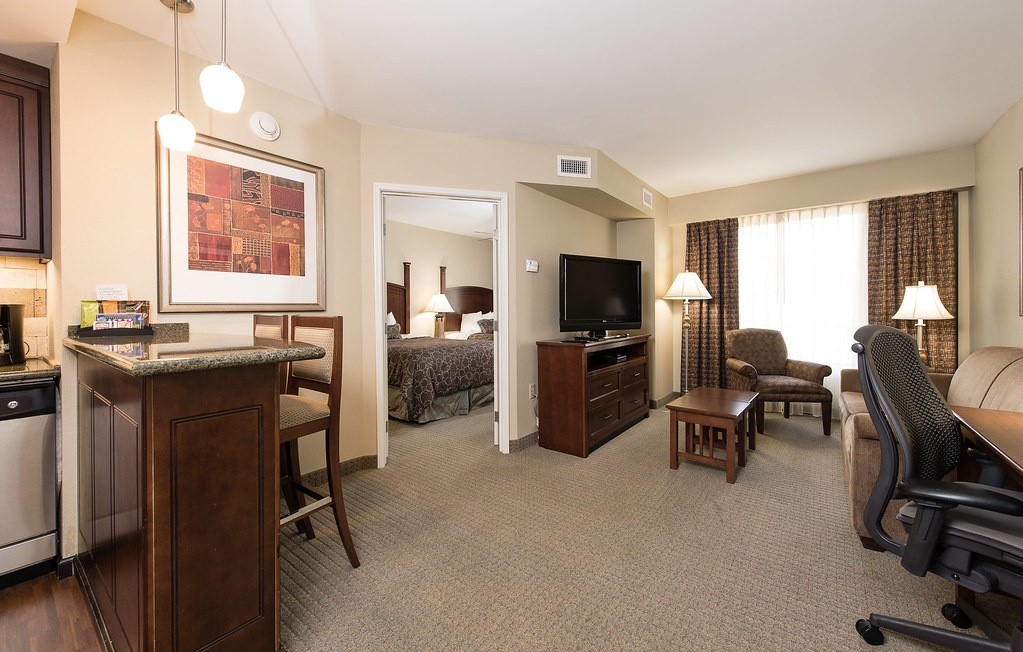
[{"xmin": 529, "ymin": 383, "xmax": 536, "ymax": 399}]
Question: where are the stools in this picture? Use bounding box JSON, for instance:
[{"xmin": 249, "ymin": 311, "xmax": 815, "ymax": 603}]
[
  {"xmin": 686, "ymin": 386, "xmax": 763, "ymax": 461},
  {"xmin": 665, "ymin": 394, "xmax": 751, "ymax": 484}
]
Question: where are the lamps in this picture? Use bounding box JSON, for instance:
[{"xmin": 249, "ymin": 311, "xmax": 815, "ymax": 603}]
[
  {"xmin": 156, "ymin": 0, "xmax": 197, "ymax": 156},
  {"xmin": 159, "ymin": 0, "xmax": 195, "ymax": 14},
  {"xmin": 661, "ymin": 269, "xmax": 714, "ymax": 393},
  {"xmin": 423, "ymin": 293, "xmax": 456, "ymax": 337},
  {"xmin": 199, "ymin": 0, "xmax": 246, "ymax": 114},
  {"xmin": 891, "ymin": 280, "xmax": 955, "ymax": 372}
]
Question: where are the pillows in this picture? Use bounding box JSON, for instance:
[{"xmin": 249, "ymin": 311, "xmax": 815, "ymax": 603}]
[
  {"xmin": 477, "ymin": 318, "xmax": 493, "ymax": 333},
  {"xmin": 387, "ymin": 311, "xmax": 397, "ymax": 325},
  {"xmin": 387, "ymin": 324, "xmax": 402, "ymax": 340},
  {"xmin": 468, "ymin": 311, "xmax": 483, "ymax": 314},
  {"xmin": 460, "ymin": 311, "xmax": 492, "ymax": 333}
]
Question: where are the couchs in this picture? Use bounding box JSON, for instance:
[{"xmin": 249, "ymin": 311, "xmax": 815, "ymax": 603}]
[{"xmin": 837, "ymin": 345, "xmax": 1023, "ymax": 554}]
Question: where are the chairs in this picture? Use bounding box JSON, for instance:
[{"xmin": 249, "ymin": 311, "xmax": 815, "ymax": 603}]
[
  {"xmin": 724, "ymin": 327, "xmax": 835, "ymax": 437},
  {"xmin": 253, "ymin": 312, "xmax": 305, "ymax": 536},
  {"xmin": 850, "ymin": 324, "xmax": 1023, "ymax": 652},
  {"xmin": 279, "ymin": 315, "xmax": 363, "ymax": 570}
]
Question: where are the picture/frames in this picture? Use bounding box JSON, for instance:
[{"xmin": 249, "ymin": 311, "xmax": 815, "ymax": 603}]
[{"xmin": 153, "ymin": 119, "xmax": 327, "ymax": 316}]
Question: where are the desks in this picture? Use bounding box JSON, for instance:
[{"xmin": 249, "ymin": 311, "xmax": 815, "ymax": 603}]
[{"xmin": 62, "ymin": 327, "xmax": 332, "ymax": 652}]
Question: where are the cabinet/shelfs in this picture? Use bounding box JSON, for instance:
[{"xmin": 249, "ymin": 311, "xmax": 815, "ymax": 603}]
[
  {"xmin": 0, "ymin": 52, "xmax": 54, "ymax": 265},
  {"xmin": 535, "ymin": 331, "xmax": 653, "ymax": 459}
]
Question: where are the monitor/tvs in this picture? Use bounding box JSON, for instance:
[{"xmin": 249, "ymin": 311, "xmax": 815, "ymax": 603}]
[{"xmin": 559, "ymin": 254, "xmax": 642, "ymax": 337}]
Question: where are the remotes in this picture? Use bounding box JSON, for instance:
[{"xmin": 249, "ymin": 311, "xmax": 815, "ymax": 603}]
[{"xmin": 561, "ymin": 340, "xmax": 590, "ymax": 343}]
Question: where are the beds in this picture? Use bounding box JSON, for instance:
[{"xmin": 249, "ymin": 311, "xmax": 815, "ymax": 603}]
[
  {"xmin": 386, "ymin": 261, "xmax": 493, "ymax": 426},
  {"xmin": 439, "ymin": 265, "xmax": 493, "ymax": 339}
]
